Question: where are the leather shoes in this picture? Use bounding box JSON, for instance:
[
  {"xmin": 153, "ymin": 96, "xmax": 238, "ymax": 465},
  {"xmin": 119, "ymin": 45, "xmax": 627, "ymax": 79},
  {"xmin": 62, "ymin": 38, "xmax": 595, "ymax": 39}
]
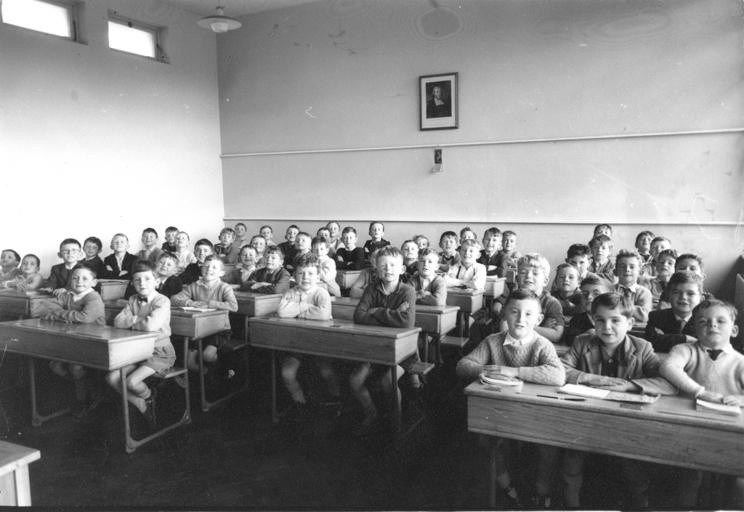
[
  {"xmin": 147, "ymin": 388, "xmax": 157, "ymax": 408},
  {"xmin": 142, "ymin": 404, "xmax": 154, "ymax": 425}
]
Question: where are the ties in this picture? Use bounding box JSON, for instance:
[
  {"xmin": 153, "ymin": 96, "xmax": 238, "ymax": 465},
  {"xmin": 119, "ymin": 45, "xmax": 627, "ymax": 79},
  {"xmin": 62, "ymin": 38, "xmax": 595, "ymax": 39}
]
[
  {"xmin": 707, "ymin": 350, "xmax": 721, "ymax": 360},
  {"xmin": 623, "ymin": 288, "xmax": 632, "ymax": 297},
  {"xmin": 678, "ymin": 320, "xmax": 686, "ymax": 329}
]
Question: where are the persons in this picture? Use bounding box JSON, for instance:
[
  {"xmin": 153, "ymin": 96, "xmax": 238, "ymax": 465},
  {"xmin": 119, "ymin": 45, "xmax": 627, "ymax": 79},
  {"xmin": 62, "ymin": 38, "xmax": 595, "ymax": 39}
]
[
  {"xmin": 170, "ymin": 253, "xmax": 238, "ymax": 380},
  {"xmin": 428, "ymin": 86, "xmax": 450, "ymax": 116},
  {"xmin": 104, "ymin": 260, "xmax": 177, "ymax": 430},
  {"xmin": 456, "ymin": 288, "xmax": 567, "ymax": 511},
  {"xmin": 3, "ymin": 223, "xmax": 717, "ymax": 352},
  {"xmin": 559, "ymin": 293, "xmax": 660, "ymax": 511},
  {"xmin": 349, "ymin": 247, "xmax": 416, "ymax": 438},
  {"xmin": 276, "ymin": 252, "xmax": 341, "ymax": 418},
  {"xmin": 660, "ymin": 299, "xmax": 744, "ymax": 512},
  {"xmin": 37, "ymin": 263, "xmax": 105, "ymax": 411}
]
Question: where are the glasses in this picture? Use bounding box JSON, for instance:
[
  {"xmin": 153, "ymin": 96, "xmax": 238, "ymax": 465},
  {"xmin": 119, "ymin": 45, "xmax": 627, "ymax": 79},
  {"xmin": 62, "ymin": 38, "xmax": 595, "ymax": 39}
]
[{"xmin": 62, "ymin": 248, "xmax": 78, "ymax": 255}]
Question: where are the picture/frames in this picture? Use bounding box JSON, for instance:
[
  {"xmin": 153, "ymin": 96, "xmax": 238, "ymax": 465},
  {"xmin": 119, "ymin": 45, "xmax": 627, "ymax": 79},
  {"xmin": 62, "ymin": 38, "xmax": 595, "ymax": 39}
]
[{"xmin": 417, "ymin": 71, "xmax": 458, "ymax": 131}]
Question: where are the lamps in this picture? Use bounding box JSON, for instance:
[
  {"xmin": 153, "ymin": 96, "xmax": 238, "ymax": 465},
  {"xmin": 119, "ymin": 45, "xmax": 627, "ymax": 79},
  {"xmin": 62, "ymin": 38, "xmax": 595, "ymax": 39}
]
[{"xmin": 196, "ymin": 0, "xmax": 242, "ymax": 34}]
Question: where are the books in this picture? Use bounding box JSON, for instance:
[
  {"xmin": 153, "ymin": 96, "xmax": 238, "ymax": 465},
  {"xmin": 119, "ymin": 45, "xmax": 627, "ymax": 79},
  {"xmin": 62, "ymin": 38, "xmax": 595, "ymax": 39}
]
[
  {"xmin": 558, "ymin": 380, "xmax": 660, "ymax": 406},
  {"xmin": 695, "ymin": 396, "xmax": 741, "ymax": 418},
  {"xmin": 478, "ymin": 372, "xmax": 524, "ymax": 393}
]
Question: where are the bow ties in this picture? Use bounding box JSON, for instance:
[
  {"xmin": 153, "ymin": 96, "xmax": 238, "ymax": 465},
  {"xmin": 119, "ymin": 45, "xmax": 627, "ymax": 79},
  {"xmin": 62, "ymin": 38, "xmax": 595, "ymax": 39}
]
[{"xmin": 138, "ymin": 296, "xmax": 147, "ymax": 302}]
